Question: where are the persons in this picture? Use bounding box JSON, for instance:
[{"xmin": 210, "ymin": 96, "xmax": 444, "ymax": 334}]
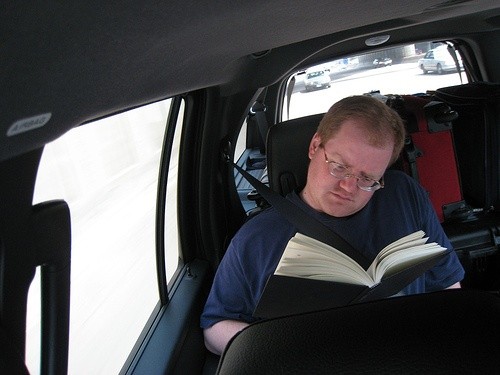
[{"xmin": 199, "ymin": 95, "xmax": 465, "ymax": 354}]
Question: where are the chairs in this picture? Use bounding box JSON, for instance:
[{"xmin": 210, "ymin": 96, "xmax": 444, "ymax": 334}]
[{"xmin": 216, "ymin": 83, "xmax": 500, "ymax": 375}]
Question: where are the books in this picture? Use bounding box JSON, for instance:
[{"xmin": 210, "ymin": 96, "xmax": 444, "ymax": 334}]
[{"xmin": 252, "ymin": 229, "xmax": 454, "ymax": 320}]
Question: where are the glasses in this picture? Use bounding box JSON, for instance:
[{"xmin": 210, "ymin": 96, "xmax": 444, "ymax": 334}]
[{"xmin": 319, "ymin": 143, "xmax": 385, "ymax": 192}]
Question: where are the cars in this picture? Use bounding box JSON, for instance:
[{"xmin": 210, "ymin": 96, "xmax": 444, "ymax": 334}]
[
  {"xmin": 373, "ymin": 57, "xmax": 392, "ymax": 67},
  {"xmin": 420, "ymin": 49, "xmax": 466, "ymax": 74},
  {"xmin": 305, "ymin": 70, "xmax": 332, "ymax": 91}
]
